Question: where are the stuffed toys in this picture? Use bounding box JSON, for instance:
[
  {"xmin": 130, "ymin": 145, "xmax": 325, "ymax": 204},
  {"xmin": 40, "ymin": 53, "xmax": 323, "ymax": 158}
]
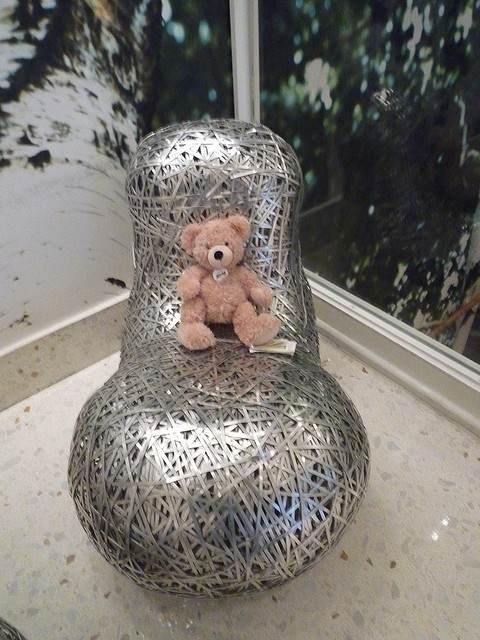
[{"xmin": 174, "ymin": 215, "xmax": 280, "ymax": 350}]
